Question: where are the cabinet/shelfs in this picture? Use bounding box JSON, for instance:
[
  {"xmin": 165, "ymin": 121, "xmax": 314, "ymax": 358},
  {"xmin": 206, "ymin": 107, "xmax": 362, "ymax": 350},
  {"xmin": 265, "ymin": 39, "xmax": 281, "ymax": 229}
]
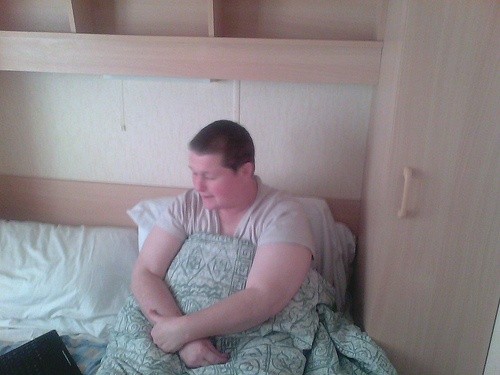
[
  {"xmin": 0, "ymin": 1, "xmax": 390, "ymax": 86},
  {"xmin": 358, "ymin": 0, "xmax": 500, "ymax": 375}
]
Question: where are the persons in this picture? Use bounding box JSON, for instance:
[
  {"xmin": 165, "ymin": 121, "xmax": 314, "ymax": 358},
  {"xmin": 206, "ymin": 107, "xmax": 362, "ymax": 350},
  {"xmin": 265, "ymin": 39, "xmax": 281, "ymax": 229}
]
[{"xmin": 130, "ymin": 120, "xmax": 316, "ymax": 369}]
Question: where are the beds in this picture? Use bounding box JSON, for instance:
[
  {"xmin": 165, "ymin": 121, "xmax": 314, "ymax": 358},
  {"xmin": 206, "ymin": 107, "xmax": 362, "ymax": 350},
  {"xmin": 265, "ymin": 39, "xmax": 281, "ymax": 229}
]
[{"xmin": 0, "ymin": 174, "xmax": 399, "ymax": 375}]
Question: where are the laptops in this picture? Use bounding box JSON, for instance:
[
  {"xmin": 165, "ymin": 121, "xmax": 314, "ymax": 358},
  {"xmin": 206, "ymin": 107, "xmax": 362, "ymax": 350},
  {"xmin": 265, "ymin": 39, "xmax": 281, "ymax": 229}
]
[{"xmin": 0, "ymin": 329, "xmax": 83, "ymax": 375}]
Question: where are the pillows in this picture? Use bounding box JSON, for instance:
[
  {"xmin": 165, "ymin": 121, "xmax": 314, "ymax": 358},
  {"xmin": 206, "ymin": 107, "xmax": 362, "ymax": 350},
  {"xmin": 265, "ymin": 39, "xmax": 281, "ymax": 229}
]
[{"xmin": 127, "ymin": 198, "xmax": 347, "ymax": 311}]
